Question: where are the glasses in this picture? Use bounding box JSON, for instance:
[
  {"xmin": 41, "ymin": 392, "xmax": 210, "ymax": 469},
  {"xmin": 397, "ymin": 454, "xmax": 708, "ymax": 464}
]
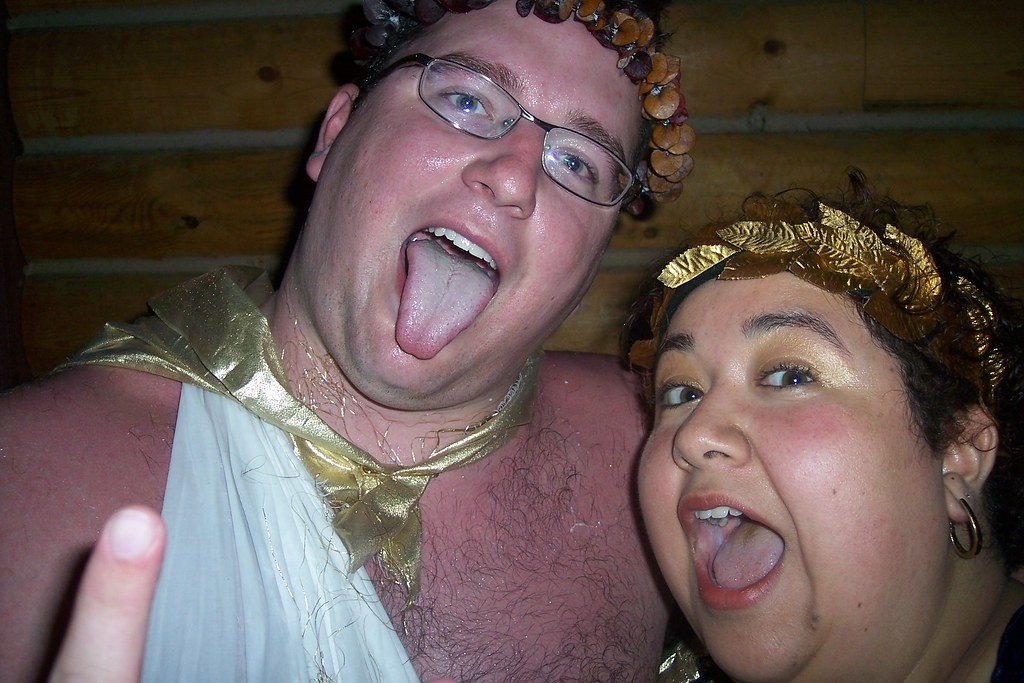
[{"xmin": 360, "ymin": 53, "xmax": 649, "ymax": 208}]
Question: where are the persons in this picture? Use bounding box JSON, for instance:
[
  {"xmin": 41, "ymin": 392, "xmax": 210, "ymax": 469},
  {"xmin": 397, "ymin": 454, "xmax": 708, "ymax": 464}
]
[
  {"xmin": 0, "ymin": 0, "xmax": 674, "ymax": 682},
  {"xmin": 620, "ymin": 186, "xmax": 1024, "ymax": 682}
]
